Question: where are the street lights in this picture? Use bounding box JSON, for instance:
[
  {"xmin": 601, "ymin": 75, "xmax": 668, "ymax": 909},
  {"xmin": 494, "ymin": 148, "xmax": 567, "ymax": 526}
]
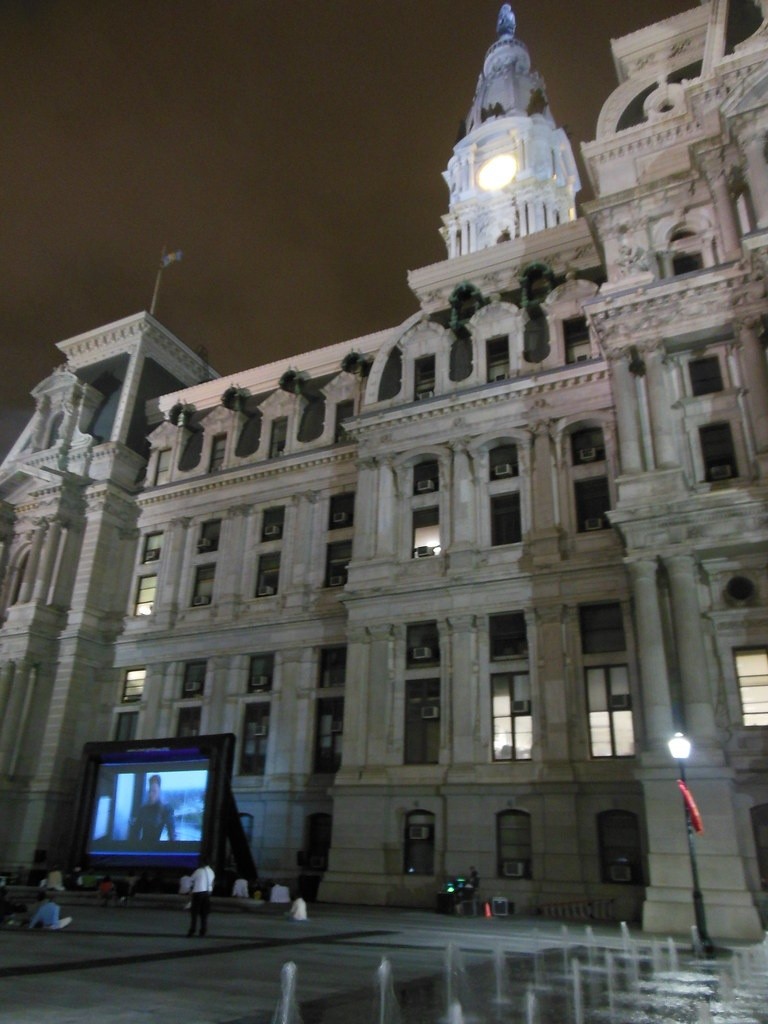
[{"xmin": 668, "ymin": 730, "xmax": 719, "ymax": 961}]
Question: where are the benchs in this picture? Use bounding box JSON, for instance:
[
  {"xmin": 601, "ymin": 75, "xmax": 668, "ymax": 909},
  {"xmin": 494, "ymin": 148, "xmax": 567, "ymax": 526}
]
[
  {"xmin": 5, "ymin": 885, "xmax": 116, "ymax": 906},
  {"xmin": 133, "ymin": 893, "xmax": 265, "ymax": 915}
]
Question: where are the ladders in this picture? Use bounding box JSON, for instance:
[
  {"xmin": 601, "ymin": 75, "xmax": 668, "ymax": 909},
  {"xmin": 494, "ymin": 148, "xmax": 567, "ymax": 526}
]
[{"xmin": 522, "ymin": 896, "xmax": 617, "ymax": 923}]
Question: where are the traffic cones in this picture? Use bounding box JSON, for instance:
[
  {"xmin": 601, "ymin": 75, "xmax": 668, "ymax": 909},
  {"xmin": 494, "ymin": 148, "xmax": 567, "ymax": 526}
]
[{"xmin": 484, "ymin": 902, "xmax": 493, "ymax": 919}]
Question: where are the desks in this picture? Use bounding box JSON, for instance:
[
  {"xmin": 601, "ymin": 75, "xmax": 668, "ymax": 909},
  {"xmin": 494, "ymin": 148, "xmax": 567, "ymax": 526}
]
[{"xmin": 437, "ymin": 887, "xmax": 464, "ymax": 914}]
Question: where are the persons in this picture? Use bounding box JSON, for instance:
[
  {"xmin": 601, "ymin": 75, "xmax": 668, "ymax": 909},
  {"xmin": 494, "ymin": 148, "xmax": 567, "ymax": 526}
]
[
  {"xmin": 125, "ymin": 775, "xmax": 177, "ymax": 841},
  {"xmin": 283, "ymin": 892, "xmax": 308, "ymax": 922},
  {"xmin": 177, "ymin": 872, "xmax": 193, "ymax": 894},
  {"xmin": 231, "ymin": 875, "xmax": 249, "ymax": 899},
  {"xmin": 269, "ymin": 878, "xmax": 289, "ymax": 902},
  {"xmin": 468, "ymin": 865, "xmax": 480, "ymax": 918},
  {"xmin": 0, "ymin": 865, "xmax": 160, "ymax": 931},
  {"xmin": 184, "ymin": 855, "xmax": 215, "ymax": 938}
]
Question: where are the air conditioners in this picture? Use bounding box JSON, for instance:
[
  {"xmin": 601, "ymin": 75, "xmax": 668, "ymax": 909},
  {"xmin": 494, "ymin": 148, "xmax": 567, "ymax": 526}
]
[
  {"xmin": 711, "ymin": 465, "xmax": 731, "ymax": 479},
  {"xmin": 197, "ymin": 539, "xmax": 212, "ymax": 548},
  {"xmin": 194, "ymin": 596, "xmax": 208, "ymax": 605},
  {"xmin": 252, "ymin": 676, "xmax": 267, "ymax": 685},
  {"xmin": 408, "ymin": 826, "xmax": 429, "ymax": 839},
  {"xmin": 259, "ymin": 587, "xmax": 273, "ymax": 595},
  {"xmin": 611, "ymin": 866, "xmax": 632, "ymax": 882},
  {"xmin": 496, "ymin": 464, "xmax": 512, "ymax": 476},
  {"xmin": 265, "ymin": 526, "xmax": 279, "ymax": 534},
  {"xmin": 330, "ymin": 576, "xmax": 344, "ymax": 585},
  {"xmin": 503, "ymin": 862, "xmax": 526, "ymax": 876},
  {"xmin": 334, "ymin": 513, "xmax": 347, "ymax": 522},
  {"xmin": 580, "ymin": 448, "xmax": 596, "ymax": 461},
  {"xmin": 422, "ymin": 706, "xmax": 439, "ymax": 718},
  {"xmin": 414, "ymin": 647, "xmax": 431, "ymax": 658},
  {"xmin": 331, "ymin": 721, "xmax": 343, "ymax": 732},
  {"xmin": 311, "ymin": 857, "xmax": 326, "ymax": 867},
  {"xmin": 418, "ymin": 480, "xmax": 434, "ymax": 490},
  {"xmin": 185, "ymin": 682, "xmax": 200, "ymax": 691},
  {"xmin": 418, "ymin": 547, "xmax": 434, "ymax": 557},
  {"xmin": 610, "ymin": 695, "xmax": 629, "ymax": 707},
  {"xmin": 586, "ymin": 518, "xmax": 603, "ymax": 529},
  {"xmin": 512, "ymin": 701, "xmax": 528, "ymax": 712}
]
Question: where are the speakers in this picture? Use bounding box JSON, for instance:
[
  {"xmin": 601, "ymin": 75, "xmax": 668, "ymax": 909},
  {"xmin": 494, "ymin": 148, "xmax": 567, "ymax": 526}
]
[{"xmin": 34, "ymin": 850, "xmax": 47, "ymax": 864}]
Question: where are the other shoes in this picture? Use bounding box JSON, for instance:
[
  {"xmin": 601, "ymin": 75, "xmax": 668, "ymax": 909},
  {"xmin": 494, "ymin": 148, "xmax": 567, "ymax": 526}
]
[
  {"xmin": 199, "ymin": 930, "xmax": 206, "ymax": 937},
  {"xmin": 185, "ymin": 928, "xmax": 195, "ymax": 938}
]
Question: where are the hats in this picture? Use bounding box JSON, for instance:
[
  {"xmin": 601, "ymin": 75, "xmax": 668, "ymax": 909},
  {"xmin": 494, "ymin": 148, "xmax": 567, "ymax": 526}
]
[{"xmin": 199, "ymin": 853, "xmax": 212, "ymax": 866}]
